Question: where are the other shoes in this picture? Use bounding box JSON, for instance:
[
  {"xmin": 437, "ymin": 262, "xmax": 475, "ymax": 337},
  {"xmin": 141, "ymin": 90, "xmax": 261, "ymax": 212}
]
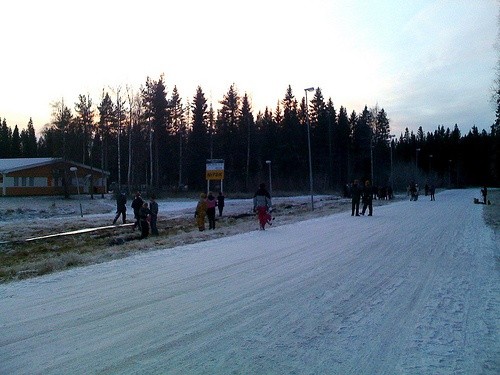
[
  {"xmin": 152, "ymin": 233, "xmax": 158, "ymax": 236},
  {"xmin": 210, "ymin": 227, "xmax": 212, "ymax": 229},
  {"xmin": 360, "ymin": 213, "xmax": 364, "ymax": 215},
  {"xmin": 140, "ymin": 236, "xmax": 145, "ymax": 239},
  {"xmin": 368, "ymin": 214, "xmax": 372, "ymax": 216},
  {"xmin": 213, "ymin": 227, "xmax": 215, "ymax": 230},
  {"xmin": 351, "ymin": 214, "xmax": 354, "ymax": 216},
  {"xmin": 356, "ymin": 214, "xmax": 360, "ymax": 216},
  {"xmin": 199, "ymin": 227, "xmax": 204, "ymax": 231}
]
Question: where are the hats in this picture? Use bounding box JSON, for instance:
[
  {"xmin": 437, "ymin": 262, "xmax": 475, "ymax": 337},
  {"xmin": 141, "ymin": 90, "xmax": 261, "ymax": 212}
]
[
  {"xmin": 137, "ymin": 192, "xmax": 141, "ymax": 196},
  {"xmin": 120, "ymin": 192, "xmax": 125, "ymax": 196},
  {"xmin": 143, "ymin": 203, "xmax": 148, "ymax": 206}
]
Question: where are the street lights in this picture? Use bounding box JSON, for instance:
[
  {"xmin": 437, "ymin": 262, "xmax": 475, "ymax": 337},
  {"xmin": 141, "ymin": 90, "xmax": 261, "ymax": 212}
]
[
  {"xmin": 370, "ymin": 145, "xmax": 373, "ymax": 186},
  {"xmin": 266, "ymin": 161, "xmax": 272, "ymax": 198},
  {"xmin": 415, "ymin": 148, "xmax": 421, "ymax": 184},
  {"xmin": 449, "ymin": 160, "xmax": 452, "ymax": 186},
  {"xmin": 305, "ymin": 88, "xmax": 314, "ymax": 211},
  {"xmin": 428, "ymin": 155, "xmax": 433, "ymax": 183},
  {"xmin": 70, "ymin": 166, "xmax": 83, "ymax": 218}
]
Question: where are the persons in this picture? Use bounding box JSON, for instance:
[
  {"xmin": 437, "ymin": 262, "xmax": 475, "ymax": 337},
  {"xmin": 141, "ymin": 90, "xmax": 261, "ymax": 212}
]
[
  {"xmin": 344, "ymin": 182, "xmax": 364, "ymax": 199},
  {"xmin": 407, "ymin": 184, "xmax": 419, "ymax": 201},
  {"xmin": 481, "ymin": 183, "xmax": 488, "ymax": 204},
  {"xmin": 217, "ymin": 192, "xmax": 224, "ymax": 217},
  {"xmin": 425, "ymin": 183, "xmax": 435, "ymax": 201},
  {"xmin": 360, "ymin": 180, "xmax": 373, "ymax": 216},
  {"xmin": 349, "ymin": 179, "xmax": 362, "ymax": 216},
  {"xmin": 131, "ymin": 192, "xmax": 144, "ymax": 230},
  {"xmin": 149, "ymin": 197, "xmax": 159, "ymax": 237},
  {"xmin": 113, "ymin": 192, "xmax": 127, "ymax": 224},
  {"xmin": 205, "ymin": 192, "xmax": 216, "ymax": 230},
  {"xmin": 194, "ymin": 193, "xmax": 207, "ymax": 231},
  {"xmin": 387, "ymin": 183, "xmax": 394, "ymax": 200},
  {"xmin": 253, "ymin": 182, "xmax": 272, "ymax": 230},
  {"xmin": 138, "ymin": 202, "xmax": 150, "ymax": 240}
]
